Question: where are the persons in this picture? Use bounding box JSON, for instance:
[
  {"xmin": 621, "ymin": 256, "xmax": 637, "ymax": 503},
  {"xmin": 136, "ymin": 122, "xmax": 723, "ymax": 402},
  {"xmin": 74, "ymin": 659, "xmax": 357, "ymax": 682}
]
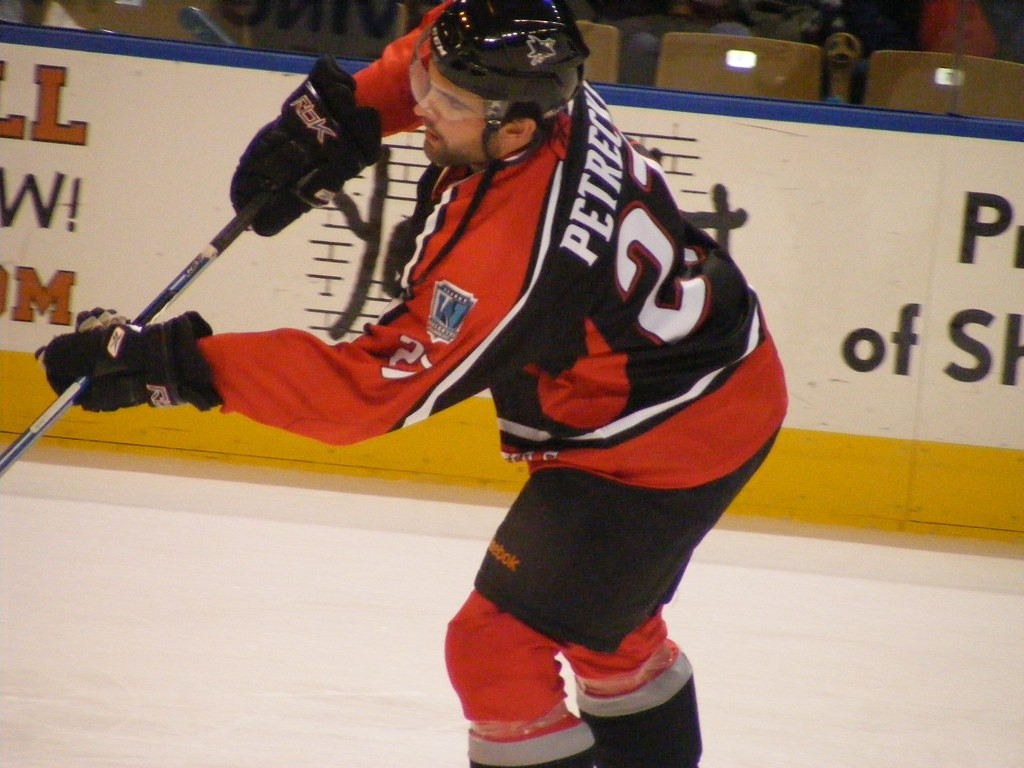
[{"xmin": 44, "ymin": 0, "xmax": 789, "ymax": 768}]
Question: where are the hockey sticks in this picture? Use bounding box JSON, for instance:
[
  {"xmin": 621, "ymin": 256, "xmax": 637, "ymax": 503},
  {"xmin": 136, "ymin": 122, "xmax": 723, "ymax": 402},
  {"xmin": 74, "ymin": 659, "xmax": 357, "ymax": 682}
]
[{"xmin": 1, "ymin": 191, "xmax": 268, "ymax": 476}]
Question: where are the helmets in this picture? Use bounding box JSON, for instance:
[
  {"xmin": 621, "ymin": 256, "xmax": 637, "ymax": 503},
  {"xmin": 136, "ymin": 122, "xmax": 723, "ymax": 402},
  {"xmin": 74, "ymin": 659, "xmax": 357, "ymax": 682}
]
[{"xmin": 428, "ymin": 0, "xmax": 592, "ymax": 125}]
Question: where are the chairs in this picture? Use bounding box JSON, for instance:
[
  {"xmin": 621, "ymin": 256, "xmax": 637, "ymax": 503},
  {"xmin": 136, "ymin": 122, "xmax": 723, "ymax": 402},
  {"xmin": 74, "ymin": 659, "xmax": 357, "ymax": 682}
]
[
  {"xmin": 655, "ymin": 33, "xmax": 820, "ymax": 104},
  {"xmin": 577, "ymin": 20, "xmax": 622, "ymax": 84},
  {"xmin": 864, "ymin": 49, "xmax": 1024, "ymax": 121},
  {"xmin": 39, "ymin": 0, "xmax": 250, "ymax": 48}
]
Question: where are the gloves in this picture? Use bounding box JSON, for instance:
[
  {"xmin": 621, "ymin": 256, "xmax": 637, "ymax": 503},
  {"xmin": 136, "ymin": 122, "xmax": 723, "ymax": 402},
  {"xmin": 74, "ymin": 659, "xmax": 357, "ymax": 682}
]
[
  {"xmin": 35, "ymin": 306, "xmax": 225, "ymax": 413},
  {"xmin": 228, "ymin": 53, "xmax": 383, "ymax": 238}
]
